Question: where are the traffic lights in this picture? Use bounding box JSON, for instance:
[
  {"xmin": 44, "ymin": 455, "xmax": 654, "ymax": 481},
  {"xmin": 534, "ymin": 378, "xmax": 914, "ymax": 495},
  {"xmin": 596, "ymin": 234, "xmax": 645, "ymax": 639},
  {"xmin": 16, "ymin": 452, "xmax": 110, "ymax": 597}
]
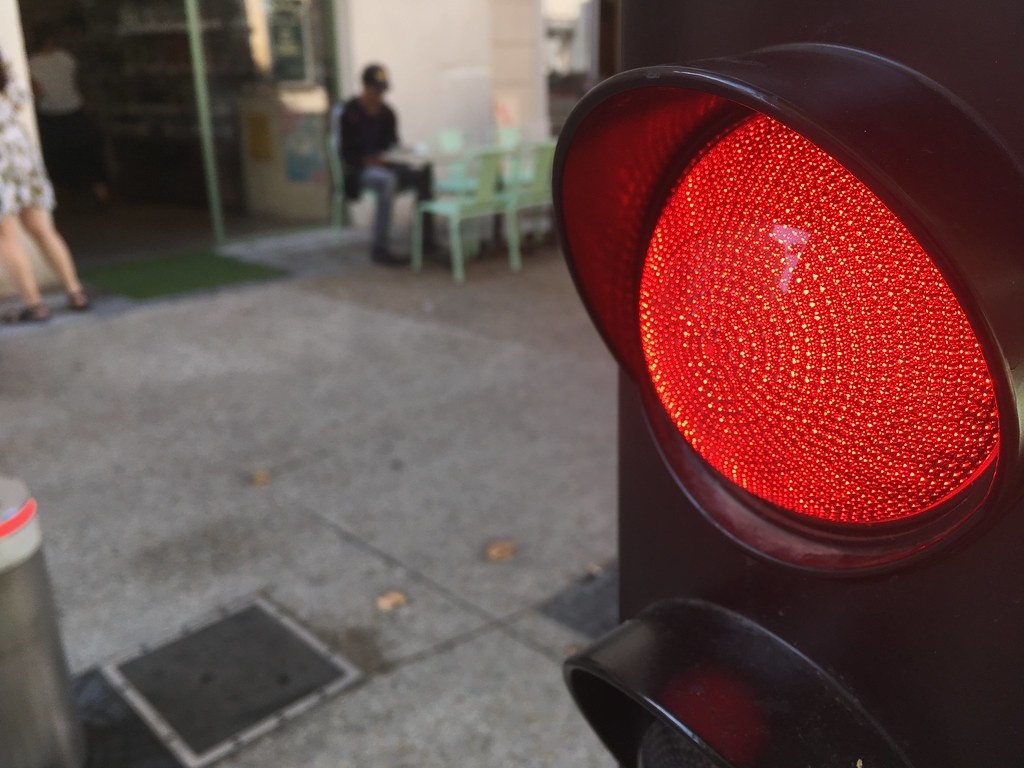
[{"xmin": 550, "ymin": 0, "xmax": 1024, "ymax": 768}]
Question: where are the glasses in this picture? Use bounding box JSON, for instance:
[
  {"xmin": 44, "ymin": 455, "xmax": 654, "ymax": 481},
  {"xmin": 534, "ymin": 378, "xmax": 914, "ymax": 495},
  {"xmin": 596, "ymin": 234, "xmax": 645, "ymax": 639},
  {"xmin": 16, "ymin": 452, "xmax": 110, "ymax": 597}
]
[{"xmin": 367, "ymin": 79, "xmax": 389, "ymax": 91}]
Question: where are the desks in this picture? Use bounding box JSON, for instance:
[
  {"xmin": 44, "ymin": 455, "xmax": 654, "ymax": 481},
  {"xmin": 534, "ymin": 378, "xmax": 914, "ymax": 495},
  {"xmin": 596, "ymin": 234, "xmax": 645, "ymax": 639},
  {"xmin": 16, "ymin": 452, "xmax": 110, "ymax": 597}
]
[{"xmin": 380, "ymin": 134, "xmax": 556, "ymax": 164}]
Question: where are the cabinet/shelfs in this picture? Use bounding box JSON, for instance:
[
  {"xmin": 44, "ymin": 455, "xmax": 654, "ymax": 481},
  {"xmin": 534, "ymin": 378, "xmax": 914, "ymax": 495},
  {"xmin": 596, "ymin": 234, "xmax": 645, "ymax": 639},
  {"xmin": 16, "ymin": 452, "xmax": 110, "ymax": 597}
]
[{"xmin": 79, "ymin": 0, "xmax": 328, "ymax": 192}]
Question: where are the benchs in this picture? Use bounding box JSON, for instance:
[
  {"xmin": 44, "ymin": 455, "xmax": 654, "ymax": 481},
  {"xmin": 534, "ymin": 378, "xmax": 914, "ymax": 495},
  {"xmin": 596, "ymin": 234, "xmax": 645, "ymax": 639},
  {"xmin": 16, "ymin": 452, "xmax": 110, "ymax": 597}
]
[{"xmin": 326, "ymin": 104, "xmax": 557, "ymax": 285}]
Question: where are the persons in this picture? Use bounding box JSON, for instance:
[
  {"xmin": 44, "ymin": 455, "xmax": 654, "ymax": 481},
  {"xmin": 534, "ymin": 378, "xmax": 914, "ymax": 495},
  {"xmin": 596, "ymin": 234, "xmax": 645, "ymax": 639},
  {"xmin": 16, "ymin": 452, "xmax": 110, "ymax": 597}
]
[
  {"xmin": 28, "ymin": 21, "xmax": 115, "ymax": 211},
  {"xmin": 0, "ymin": 53, "xmax": 92, "ymax": 323},
  {"xmin": 338, "ymin": 64, "xmax": 445, "ymax": 268}
]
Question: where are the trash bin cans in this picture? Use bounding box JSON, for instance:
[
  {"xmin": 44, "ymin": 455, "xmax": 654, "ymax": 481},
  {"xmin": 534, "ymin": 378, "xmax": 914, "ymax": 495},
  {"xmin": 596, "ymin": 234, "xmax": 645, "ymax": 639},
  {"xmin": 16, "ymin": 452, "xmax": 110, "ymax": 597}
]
[{"xmin": 0, "ymin": 474, "xmax": 104, "ymax": 768}]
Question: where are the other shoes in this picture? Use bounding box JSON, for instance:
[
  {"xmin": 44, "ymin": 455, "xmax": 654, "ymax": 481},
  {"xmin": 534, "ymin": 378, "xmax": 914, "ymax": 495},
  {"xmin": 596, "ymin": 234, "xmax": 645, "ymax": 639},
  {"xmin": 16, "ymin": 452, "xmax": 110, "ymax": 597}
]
[
  {"xmin": 420, "ymin": 243, "xmax": 450, "ymax": 260},
  {"xmin": 2, "ymin": 302, "xmax": 51, "ymax": 323},
  {"xmin": 67, "ymin": 288, "xmax": 90, "ymax": 310},
  {"xmin": 370, "ymin": 247, "xmax": 410, "ymax": 266}
]
